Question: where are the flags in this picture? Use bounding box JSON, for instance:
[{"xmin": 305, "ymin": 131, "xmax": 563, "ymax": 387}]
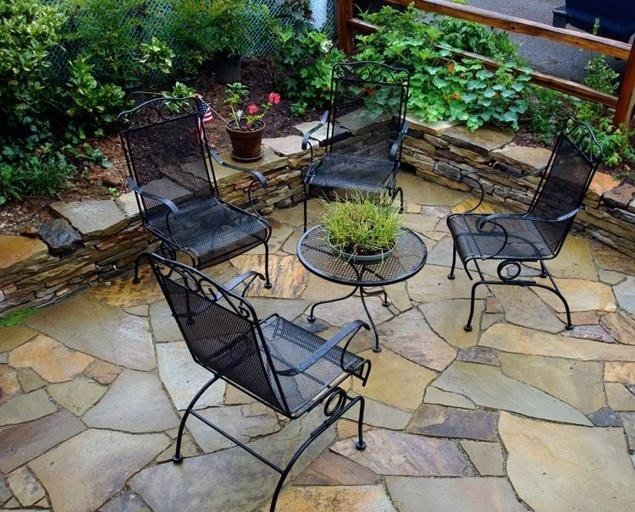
[{"xmin": 194, "ymin": 96, "xmax": 213, "ymax": 141}]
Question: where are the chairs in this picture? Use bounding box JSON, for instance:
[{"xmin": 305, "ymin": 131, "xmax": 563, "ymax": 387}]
[
  {"xmin": 130, "ymin": 250, "xmax": 373, "ymax": 512},
  {"xmin": 445, "ymin": 115, "xmax": 602, "ymax": 333},
  {"xmin": 300, "ymin": 60, "xmax": 410, "ymax": 241},
  {"xmin": 118, "ymin": 94, "xmax": 274, "ymax": 327}
]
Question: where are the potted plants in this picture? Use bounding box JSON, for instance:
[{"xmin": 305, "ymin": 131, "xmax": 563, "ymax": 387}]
[{"xmin": 310, "ymin": 181, "xmax": 410, "ymax": 275}]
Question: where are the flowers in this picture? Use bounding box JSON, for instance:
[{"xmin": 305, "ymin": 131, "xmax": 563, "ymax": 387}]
[{"xmin": 223, "ymin": 82, "xmax": 280, "ymax": 131}]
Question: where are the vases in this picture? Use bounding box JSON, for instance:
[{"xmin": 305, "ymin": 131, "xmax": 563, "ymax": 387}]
[{"xmin": 226, "ymin": 117, "xmax": 266, "ymax": 162}]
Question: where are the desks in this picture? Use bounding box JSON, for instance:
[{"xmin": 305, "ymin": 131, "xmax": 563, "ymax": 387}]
[{"xmin": 296, "ymin": 222, "xmax": 428, "ymax": 354}]
